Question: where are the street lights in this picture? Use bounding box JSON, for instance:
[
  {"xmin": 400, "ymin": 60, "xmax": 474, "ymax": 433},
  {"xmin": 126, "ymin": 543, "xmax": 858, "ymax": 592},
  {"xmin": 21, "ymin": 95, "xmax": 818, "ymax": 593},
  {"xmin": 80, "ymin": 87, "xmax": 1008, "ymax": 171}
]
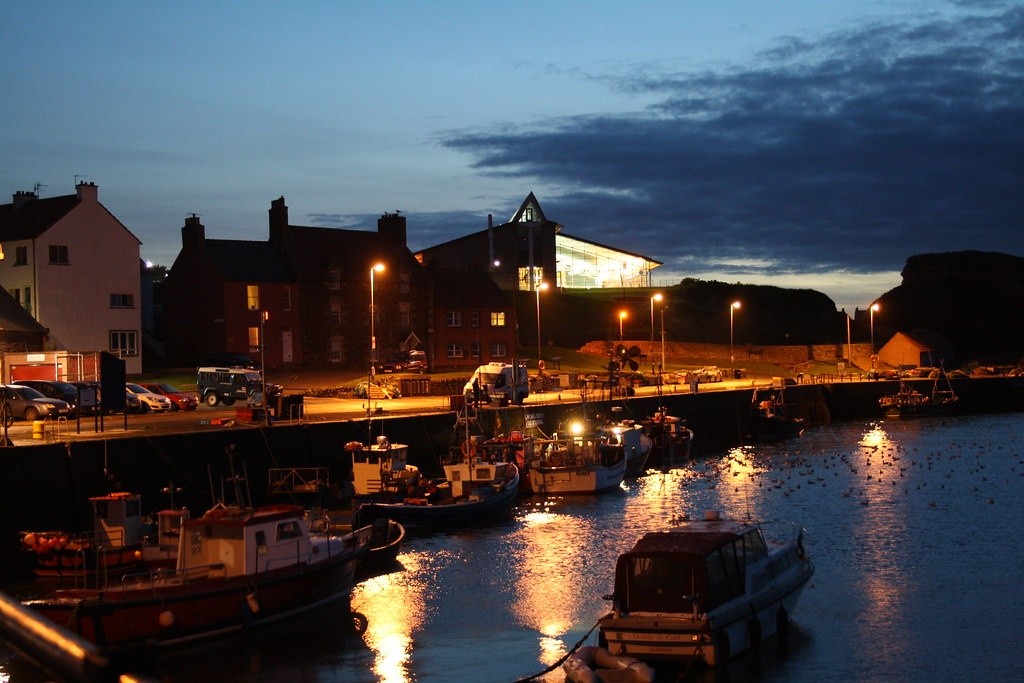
[
  {"xmin": 259, "ymin": 307, "xmax": 270, "ymax": 423},
  {"xmin": 650, "ymin": 293, "xmax": 664, "ymax": 371},
  {"xmin": 370, "ymin": 261, "xmax": 386, "ymax": 380},
  {"xmin": 870, "ymin": 302, "xmax": 880, "ymax": 370},
  {"xmin": 619, "ymin": 309, "xmax": 629, "ymax": 370},
  {"xmin": 730, "ymin": 300, "xmax": 742, "ymax": 374},
  {"xmin": 536, "ymin": 282, "xmax": 549, "ymax": 361}
]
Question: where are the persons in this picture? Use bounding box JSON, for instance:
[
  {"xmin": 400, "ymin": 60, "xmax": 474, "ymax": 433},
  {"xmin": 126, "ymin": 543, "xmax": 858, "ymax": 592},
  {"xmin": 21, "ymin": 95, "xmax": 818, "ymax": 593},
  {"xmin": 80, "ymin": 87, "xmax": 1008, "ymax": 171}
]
[
  {"xmin": 690, "ymin": 370, "xmax": 700, "ymax": 392},
  {"xmin": 472, "ymin": 378, "xmax": 479, "ymax": 407}
]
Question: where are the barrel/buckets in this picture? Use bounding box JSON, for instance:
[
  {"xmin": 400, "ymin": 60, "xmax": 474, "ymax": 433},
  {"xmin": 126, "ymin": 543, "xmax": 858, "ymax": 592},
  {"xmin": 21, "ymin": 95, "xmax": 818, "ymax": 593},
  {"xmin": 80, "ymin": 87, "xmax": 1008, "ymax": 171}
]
[
  {"xmin": 690, "ymin": 381, "xmax": 698, "ymax": 392},
  {"xmin": 274, "ymin": 394, "xmax": 303, "ymax": 420},
  {"xmin": 671, "ymin": 385, "xmax": 676, "ymax": 393},
  {"xmin": 33, "ymin": 420, "xmax": 44, "ymax": 439}
]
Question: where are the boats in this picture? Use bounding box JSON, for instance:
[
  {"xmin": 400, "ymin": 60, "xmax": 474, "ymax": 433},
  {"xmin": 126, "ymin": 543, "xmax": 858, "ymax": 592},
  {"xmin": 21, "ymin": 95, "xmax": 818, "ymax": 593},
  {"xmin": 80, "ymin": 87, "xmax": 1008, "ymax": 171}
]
[
  {"xmin": 15, "ymin": 418, "xmax": 360, "ymax": 655},
  {"xmin": 562, "ymin": 643, "xmax": 657, "ymax": 683},
  {"xmin": 322, "ymin": 515, "xmax": 406, "ymax": 571},
  {"xmin": 876, "ymin": 353, "xmax": 961, "ymax": 419},
  {"xmin": 598, "ymin": 477, "xmax": 818, "ymax": 668},
  {"xmin": 443, "ymin": 357, "xmax": 695, "ymax": 495},
  {"xmin": 740, "ymin": 381, "xmax": 812, "ymax": 440},
  {"xmin": 343, "ymin": 389, "xmax": 521, "ymax": 530}
]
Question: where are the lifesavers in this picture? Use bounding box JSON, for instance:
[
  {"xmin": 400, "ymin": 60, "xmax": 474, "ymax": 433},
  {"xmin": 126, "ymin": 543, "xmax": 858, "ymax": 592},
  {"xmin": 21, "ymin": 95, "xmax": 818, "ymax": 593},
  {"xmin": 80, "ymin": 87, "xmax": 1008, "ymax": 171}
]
[
  {"xmin": 538, "ymin": 360, "xmax": 545, "ymax": 370},
  {"xmin": 871, "ymin": 355, "xmax": 876, "ymax": 361},
  {"xmin": 461, "ymin": 439, "xmax": 476, "ymax": 457},
  {"xmin": 109, "ymin": 493, "xmax": 131, "ymax": 497}
]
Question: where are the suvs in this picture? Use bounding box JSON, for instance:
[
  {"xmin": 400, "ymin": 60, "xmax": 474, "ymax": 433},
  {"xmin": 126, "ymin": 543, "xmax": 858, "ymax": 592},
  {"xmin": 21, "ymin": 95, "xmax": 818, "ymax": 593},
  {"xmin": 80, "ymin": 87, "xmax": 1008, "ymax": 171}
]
[{"xmin": 196, "ymin": 365, "xmax": 276, "ymax": 408}]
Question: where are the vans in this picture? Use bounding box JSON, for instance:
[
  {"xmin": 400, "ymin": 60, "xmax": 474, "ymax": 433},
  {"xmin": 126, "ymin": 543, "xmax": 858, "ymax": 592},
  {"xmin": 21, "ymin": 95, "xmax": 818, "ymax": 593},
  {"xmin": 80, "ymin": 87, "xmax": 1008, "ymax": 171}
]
[{"xmin": 462, "ymin": 362, "xmax": 530, "ymax": 407}]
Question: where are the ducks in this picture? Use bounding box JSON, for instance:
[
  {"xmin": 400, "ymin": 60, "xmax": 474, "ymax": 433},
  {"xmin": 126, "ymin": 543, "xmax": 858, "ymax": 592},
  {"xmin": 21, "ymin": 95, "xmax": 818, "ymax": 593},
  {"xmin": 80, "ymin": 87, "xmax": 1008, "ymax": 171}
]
[{"xmin": 668, "ymin": 429, "xmax": 1024, "ymax": 508}]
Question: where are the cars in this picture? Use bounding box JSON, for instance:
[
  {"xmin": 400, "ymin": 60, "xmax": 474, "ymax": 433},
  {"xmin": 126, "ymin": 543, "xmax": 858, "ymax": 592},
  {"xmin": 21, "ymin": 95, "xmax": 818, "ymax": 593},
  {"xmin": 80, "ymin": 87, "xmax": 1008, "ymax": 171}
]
[
  {"xmin": 125, "ymin": 382, "xmax": 172, "ymax": 413},
  {"xmin": 10, "ymin": 379, "xmax": 143, "ymax": 417},
  {"xmin": 138, "ymin": 381, "xmax": 201, "ymax": 411},
  {"xmin": 0, "ymin": 383, "xmax": 72, "ymax": 421}
]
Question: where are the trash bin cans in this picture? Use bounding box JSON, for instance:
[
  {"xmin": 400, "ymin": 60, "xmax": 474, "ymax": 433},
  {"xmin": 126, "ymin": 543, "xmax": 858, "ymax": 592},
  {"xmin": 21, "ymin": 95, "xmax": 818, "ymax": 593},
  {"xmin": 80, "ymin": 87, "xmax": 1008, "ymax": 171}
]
[
  {"xmin": 690, "ymin": 381, "xmax": 698, "ymax": 392},
  {"xmin": 735, "ymin": 370, "xmax": 741, "ymax": 379},
  {"xmin": 275, "ymin": 394, "xmax": 303, "ymax": 419}
]
